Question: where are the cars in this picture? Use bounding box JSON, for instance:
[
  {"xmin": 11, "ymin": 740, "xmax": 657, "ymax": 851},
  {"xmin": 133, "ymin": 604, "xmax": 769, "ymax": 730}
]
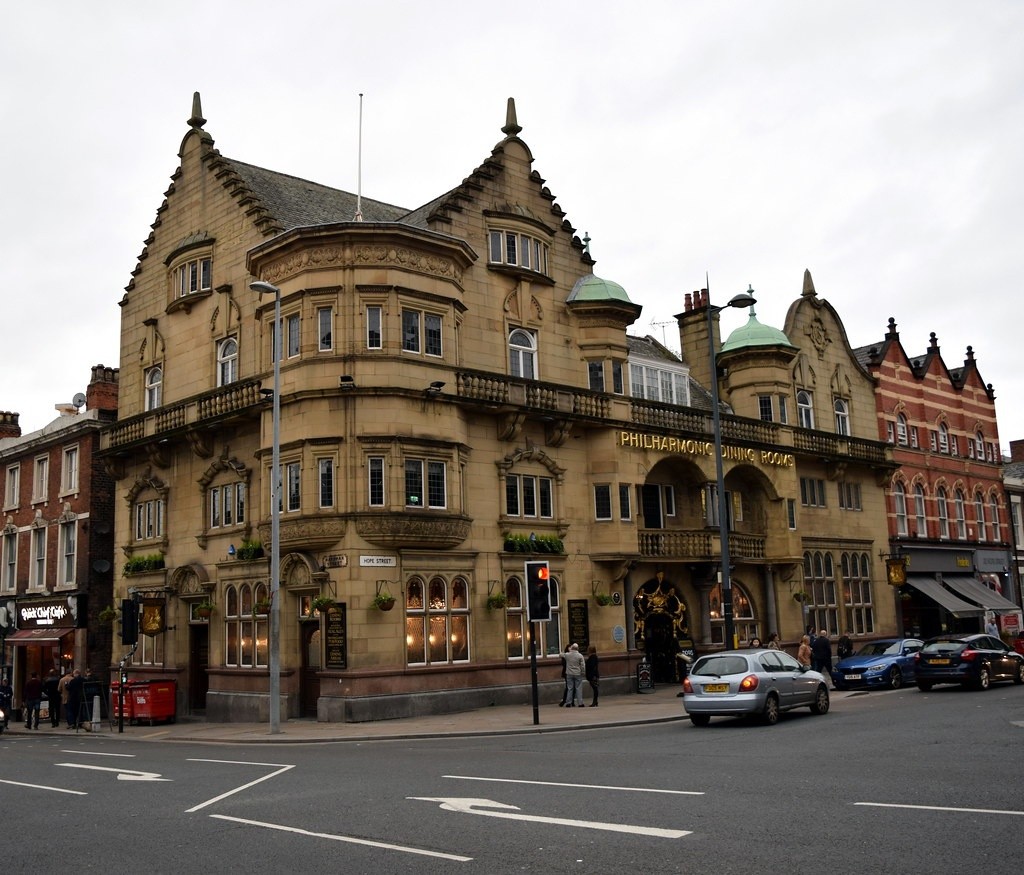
[
  {"xmin": 683, "ymin": 649, "xmax": 831, "ymax": 728},
  {"xmin": 830, "ymin": 638, "xmax": 924, "ymax": 690},
  {"xmin": 915, "ymin": 633, "xmax": 1024, "ymax": 690}
]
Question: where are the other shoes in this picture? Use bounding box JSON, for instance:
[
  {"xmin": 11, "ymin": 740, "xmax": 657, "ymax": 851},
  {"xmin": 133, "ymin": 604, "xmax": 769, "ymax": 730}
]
[
  {"xmin": 5, "ymin": 724, "xmax": 9, "ymax": 729},
  {"xmin": 559, "ymin": 701, "xmax": 565, "ymax": 707},
  {"xmin": 566, "ymin": 704, "xmax": 571, "ymax": 707},
  {"xmin": 53, "ymin": 723, "xmax": 58, "ymax": 728},
  {"xmin": 67, "ymin": 723, "xmax": 74, "ymax": 729},
  {"xmin": 34, "ymin": 726, "xmax": 38, "ymax": 730},
  {"xmin": 25, "ymin": 724, "xmax": 31, "ymax": 729},
  {"xmin": 579, "ymin": 704, "xmax": 585, "ymax": 707},
  {"xmin": 572, "ymin": 703, "xmax": 576, "ymax": 706}
]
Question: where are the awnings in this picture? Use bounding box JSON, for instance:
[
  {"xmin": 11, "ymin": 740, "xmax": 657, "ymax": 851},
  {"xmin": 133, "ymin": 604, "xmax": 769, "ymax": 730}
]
[
  {"xmin": 5, "ymin": 628, "xmax": 75, "ymax": 647},
  {"xmin": 942, "ymin": 575, "xmax": 1021, "ymax": 611},
  {"xmin": 907, "ymin": 575, "xmax": 985, "ymax": 619}
]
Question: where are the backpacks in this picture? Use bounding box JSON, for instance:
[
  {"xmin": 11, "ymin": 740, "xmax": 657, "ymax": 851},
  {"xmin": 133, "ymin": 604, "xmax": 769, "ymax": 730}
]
[{"xmin": 837, "ymin": 638, "xmax": 847, "ymax": 657}]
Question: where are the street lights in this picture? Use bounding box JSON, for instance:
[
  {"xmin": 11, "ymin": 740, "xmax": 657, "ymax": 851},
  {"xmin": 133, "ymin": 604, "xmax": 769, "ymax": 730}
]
[
  {"xmin": 704, "ymin": 295, "xmax": 758, "ymax": 651},
  {"xmin": 250, "ymin": 279, "xmax": 284, "ymax": 735}
]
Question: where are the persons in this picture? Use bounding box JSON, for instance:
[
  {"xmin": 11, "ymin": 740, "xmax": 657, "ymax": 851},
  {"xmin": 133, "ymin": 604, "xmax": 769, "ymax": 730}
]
[
  {"xmin": 0, "ymin": 678, "xmax": 13, "ymax": 729},
  {"xmin": 43, "ymin": 669, "xmax": 62, "ymax": 728},
  {"xmin": 798, "ymin": 625, "xmax": 832, "ymax": 676},
  {"xmin": 559, "ymin": 643, "xmax": 586, "ymax": 708},
  {"xmin": 585, "ymin": 645, "xmax": 600, "ymax": 706},
  {"xmin": 768, "ymin": 632, "xmax": 782, "ymax": 650},
  {"xmin": 23, "ymin": 672, "xmax": 42, "ymax": 730},
  {"xmin": 837, "ymin": 629, "xmax": 853, "ymax": 660},
  {"xmin": 749, "ymin": 638, "xmax": 763, "ymax": 649},
  {"xmin": 58, "ymin": 668, "xmax": 98, "ymax": 730},
  {"xmin": 987, "ymin": 618, "xmax": 999, "ymax": 639}
]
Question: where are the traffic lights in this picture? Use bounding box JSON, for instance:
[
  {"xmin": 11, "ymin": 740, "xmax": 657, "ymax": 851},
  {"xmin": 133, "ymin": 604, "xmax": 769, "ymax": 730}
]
[
  {"xmin": 523, "ymin": 560, "xmax": 552, "ymax": 622},
  {"xmin": 117, "ymin": 599, "xmax": 136, "ymax": 645}
]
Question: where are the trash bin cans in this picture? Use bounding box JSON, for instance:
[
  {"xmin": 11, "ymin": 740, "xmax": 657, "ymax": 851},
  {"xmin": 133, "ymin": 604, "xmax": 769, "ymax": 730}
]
[
  {"xmin": 129, "ymin": 679, "xmax": 177, "ymax": 727},
  {"xmin": 110, "ymin": 679, "xmax": 144, "ymax": 726}
]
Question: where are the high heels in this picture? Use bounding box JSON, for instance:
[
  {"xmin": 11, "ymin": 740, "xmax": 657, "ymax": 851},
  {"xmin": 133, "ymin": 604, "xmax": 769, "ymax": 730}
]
[{"xmin": 589, "ymin": 702, "xmax": 598, "ymax": 707}]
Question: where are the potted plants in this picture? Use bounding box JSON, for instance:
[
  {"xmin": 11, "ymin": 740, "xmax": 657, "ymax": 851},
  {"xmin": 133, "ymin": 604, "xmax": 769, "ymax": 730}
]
[
  {"xmin": 488, "ymin": 578, "xmax": 507, "ymax": 609},
  {"xmin": 253, "ymin": 596, "xmax": 271, "ymax": 620},
  {"xmin": 502, "ymin": 531, "xmax": 564, "ymax": 554},
  {"xmin": 306, "ymin": 581, "xmax": 339, "ymax": 620},
  {"xmin": 195, "ymin": 600, "xmax": 211, "ymax": 618},
  {"xmin": 121, "ymin": 554, "xmax": 165, "ymax": 573},
  {"xmin": 236, "ymin": 539, "xmax": 265, "ymax": 560},
  {"xmin": 594, "ymin": 580, "xmax": 611, "ymax": 606},
  {"xmin": 795, "ymin": 577, "xmax": 809, "ymax": 602},
  {"xmin": 370, "ymin": 579, "xmax": 397, "ymax": 611}
]
